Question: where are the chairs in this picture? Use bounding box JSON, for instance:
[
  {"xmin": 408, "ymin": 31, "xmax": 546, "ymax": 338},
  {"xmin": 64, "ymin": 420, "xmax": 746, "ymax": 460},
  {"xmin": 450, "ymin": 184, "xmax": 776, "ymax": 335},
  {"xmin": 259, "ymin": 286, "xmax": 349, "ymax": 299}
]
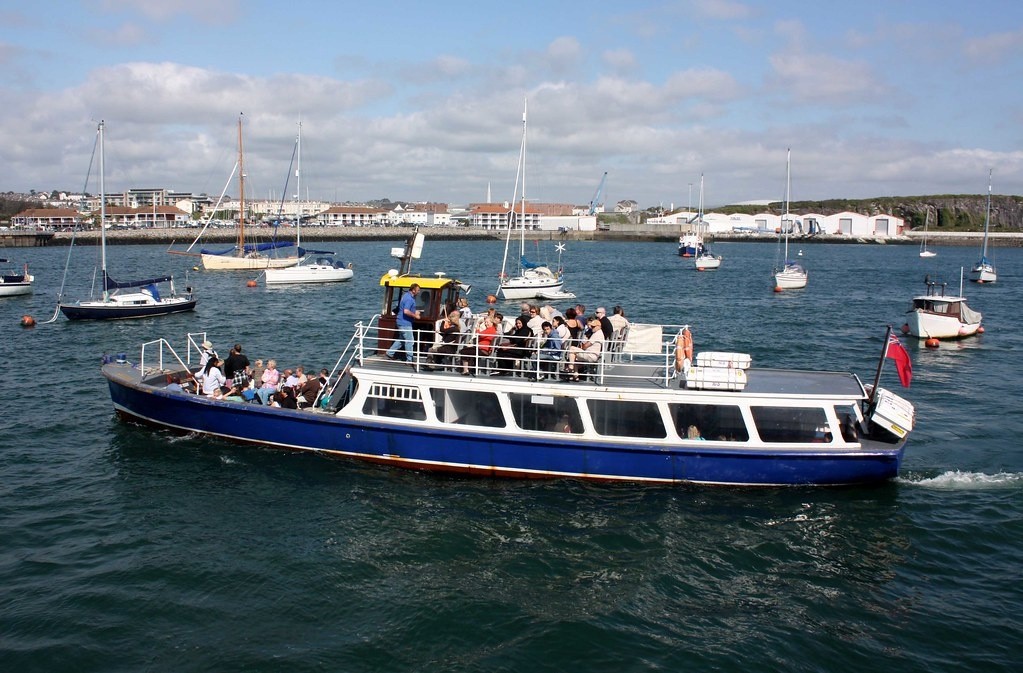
[{"xmin": 430, "ymin": 313, "xmax": 628, "ymax": 383}]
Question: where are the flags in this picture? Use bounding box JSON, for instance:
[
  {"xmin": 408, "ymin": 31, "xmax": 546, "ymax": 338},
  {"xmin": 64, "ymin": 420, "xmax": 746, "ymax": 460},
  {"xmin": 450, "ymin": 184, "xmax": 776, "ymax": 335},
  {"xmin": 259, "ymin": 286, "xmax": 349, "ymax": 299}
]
[{"xmin": 886, "ymin": 331, "xmax": 911, "ymax": 386}]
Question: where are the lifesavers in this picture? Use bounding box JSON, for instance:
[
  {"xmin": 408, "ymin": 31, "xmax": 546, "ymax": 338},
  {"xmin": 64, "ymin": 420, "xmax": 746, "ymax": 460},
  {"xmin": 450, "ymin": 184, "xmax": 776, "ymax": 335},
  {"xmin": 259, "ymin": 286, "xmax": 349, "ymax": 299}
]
[
  {"xmin": 675, "ymin": 336, "xmax": 685, "ymax": 372},
  {"xmin": 682, "ymin": 329, "xmax": 693, "ymax": 363}
]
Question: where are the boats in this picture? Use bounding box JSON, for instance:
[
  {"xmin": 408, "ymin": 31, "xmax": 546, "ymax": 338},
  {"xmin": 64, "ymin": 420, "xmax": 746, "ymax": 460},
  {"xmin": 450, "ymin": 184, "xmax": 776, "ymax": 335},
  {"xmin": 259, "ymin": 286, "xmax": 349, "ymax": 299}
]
[
  {"xmin": 100, "ymin": 220, "xmax": 917, "ymax": 493},
  {"xmin": 535, "ymin": 288, "xmax": 577, "ymax": 301},
  {"xmin": 900, "ymin": 267, "xmax": 986, "ymax": 348},
  {"xmin": 678, "ymin": 219, "xmax": 710, "ymax": 258},
  {"xmin": 0, "ymin": 258, "xmax": 35, "ymax": 299}
]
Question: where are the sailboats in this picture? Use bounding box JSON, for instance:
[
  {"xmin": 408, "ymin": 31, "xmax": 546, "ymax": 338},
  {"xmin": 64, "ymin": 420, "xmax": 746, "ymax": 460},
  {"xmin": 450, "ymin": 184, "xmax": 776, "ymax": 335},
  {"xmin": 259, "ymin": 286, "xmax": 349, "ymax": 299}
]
[
  {"xmin": 164, "ymin": 108, "xmax": 307, "ymax": 271},
  {"xmin": 20, "ymin": 117, "xmax": 201, "ymax": 329},
  {"xmin": 966, "ymin": 168, "xmax": 998, "ymax": 284},
  {"xmin": 693, "ymin": 173, "xmax": 723, "ymax": 272},
  {"xmin": 770, "ymin": 146, "xmax": 809, "ymax": 292},
  {"xmin": 485, "ymin": 97, "xmax": 566, "ymax": 304},
  {"xmin": 919, "ymin": 207, "xmax": 938, "ymax": 258},
  {"xmin": 246, "ymin": 121, "xmax": 355, "ymax": 287}
]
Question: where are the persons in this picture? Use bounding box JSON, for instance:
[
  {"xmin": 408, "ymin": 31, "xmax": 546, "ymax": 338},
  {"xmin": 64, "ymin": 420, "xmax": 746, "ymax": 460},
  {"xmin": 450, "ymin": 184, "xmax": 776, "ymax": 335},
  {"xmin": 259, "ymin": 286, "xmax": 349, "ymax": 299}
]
[
  {"xmin": 530, "ymin": 321, "xmax": 562, "ymax": 380},
  {"xmin": 268, "ymin": 367, "xmax": 345, "ymax": 410},
  {"xmin": 490, "ymin": 316, "xmax": 533, "ymax": 375},
  {"xmin": 553, "ymin": 414, "xmax": 571, "ymax": 433},
  {"xmin": 575, "ymin": 305, "xmax": 587, "ymax": 326},
  {"xmin": 518, "ymin": 304, "xmax": 564, "ymax": 348},
  {"xmin": 256, "ymin": 359, "xmax": 279, "ymax": 405},
  {"xmin": 421, "ymin": 311, "xmax": 468, "ymax": 371},
  {"xmin": 584, "ymin": 307, "xmax": 613, "ymax": 339},
  {"xmin": 386, "ymin": 283, "xmax": 421, "ymax": 366},
  {"xmin": 687, "ymin": 425, "xmax": 705, "ymax": 440},
  {"xmin": 564, "ymin": 308, "xmax": 584, "ymax": 346},
  {"xmin": 195, "ymin": 341, "xmax": 227, "ymax": 396},
  {"xmin": 457, "ymin": 299, "xmax": 472, "ymax": 318},
  {"xmin": 212, "ymin": 345, "xmax": 264, "ymax": 403},
  {"xmin": 607, "ymin": 306, "xmax": 629, "ymax": 338},
  {"xmin": 552, "ymin": 317, "xmax": 570, "ymax": 343},
  {"xmin": 459, "ymin": 308, "xmax": 503, "ymax": 376},
  {"xmin": 559, "ymin": 320, "xmax": 604, "ymax": 382}
]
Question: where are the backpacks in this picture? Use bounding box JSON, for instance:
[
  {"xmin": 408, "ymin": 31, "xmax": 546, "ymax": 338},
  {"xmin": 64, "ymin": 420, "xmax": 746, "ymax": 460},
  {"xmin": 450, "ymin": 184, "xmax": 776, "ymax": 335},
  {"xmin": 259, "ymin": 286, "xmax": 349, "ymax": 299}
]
[{"xmin": 207, "ymin": 350, "xmax": 216, "ymax": 359}]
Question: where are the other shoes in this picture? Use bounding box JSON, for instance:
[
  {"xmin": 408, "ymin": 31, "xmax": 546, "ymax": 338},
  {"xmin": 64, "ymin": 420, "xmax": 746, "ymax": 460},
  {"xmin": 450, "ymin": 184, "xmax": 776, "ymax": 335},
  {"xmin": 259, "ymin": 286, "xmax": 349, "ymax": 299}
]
[
  {"xmin": 405, "ymin": 363, "xmax": 414, "ymax": 367},
  {"xmin": 528, "ymin": 373, "xmax": 545, "ymax": 382},
  {"xmin": 420, "ymin": 365, "xmax": 436, "ymax": 371},
  {"xmin": 489, "ymin": 370, "xmax": 501, "ymax": 376},
  {"xmin": 461, "ymin": 371, "xmax": 470, "ymax": 376},
  {"xmin": 385, "ymin": 353, "xmax": 397, "ymax": 360}
]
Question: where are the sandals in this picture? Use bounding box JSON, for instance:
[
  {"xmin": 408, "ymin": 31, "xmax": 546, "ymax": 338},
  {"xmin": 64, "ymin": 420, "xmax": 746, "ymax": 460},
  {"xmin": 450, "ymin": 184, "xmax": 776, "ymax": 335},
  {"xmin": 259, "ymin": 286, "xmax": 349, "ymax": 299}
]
[
  {"xmin": 561, "ymin": 366, "xmax": 574, "ymax": 372},
  {"xmin": 566, "ymin": 375, "xmax": 580, "ymax": 381}
]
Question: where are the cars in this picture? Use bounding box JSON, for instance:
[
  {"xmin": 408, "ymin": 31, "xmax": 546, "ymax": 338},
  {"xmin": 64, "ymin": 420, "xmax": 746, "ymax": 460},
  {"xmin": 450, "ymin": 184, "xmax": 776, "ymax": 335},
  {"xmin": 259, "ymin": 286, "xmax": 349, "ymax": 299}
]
[
  {"xmin": 10, "ymin": 223, "xmax": 96, "ymax": 232},
  {"xmin": 170, "ymin": 217, "xmax": 469, "ymax": 228}
]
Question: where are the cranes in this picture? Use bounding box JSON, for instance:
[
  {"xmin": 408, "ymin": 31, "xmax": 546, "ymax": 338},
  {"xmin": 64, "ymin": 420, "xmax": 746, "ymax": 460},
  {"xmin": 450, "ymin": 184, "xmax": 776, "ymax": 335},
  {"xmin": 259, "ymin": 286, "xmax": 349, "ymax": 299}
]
[{"xmin": 585, "ymin": 170, "xmax": 609, "ymax": 217}]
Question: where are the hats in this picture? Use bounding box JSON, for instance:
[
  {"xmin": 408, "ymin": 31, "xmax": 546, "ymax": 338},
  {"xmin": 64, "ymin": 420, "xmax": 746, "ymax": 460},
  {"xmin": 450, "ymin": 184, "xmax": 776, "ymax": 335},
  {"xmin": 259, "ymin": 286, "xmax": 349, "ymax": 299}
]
[
  {"xmin": 588, "ymin": 319, "xmax": 601, "ymax": 326},
  {"xmin": 305, "ymin": 370, "xmax": 315, "ymax": 375},
  {"xmin": 201, "ymin": 341, "xmax": 213, "ymax": 349}
]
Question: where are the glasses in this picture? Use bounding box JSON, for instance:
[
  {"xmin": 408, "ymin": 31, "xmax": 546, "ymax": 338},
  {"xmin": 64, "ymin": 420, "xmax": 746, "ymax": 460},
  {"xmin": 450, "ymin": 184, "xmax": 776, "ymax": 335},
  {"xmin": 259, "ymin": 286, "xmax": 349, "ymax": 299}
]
[
  {"xmin": 530, "ymin": 311, "xmax": 535, "ymax": 313},
  {"xmin": 596, "ymin": 312, "xmax": 602, "ymax": 314}
]
